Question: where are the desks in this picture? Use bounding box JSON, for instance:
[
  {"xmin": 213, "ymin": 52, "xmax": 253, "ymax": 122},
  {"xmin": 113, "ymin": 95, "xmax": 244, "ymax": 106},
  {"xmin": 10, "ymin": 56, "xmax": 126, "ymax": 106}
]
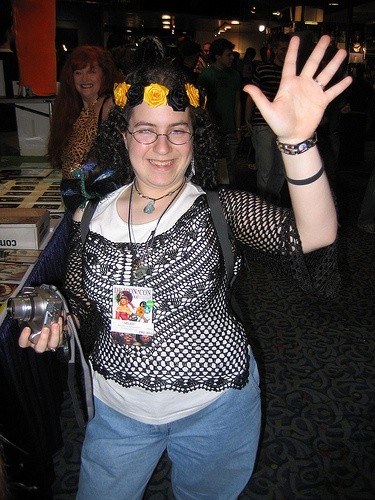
[{"xmin": 0, "ymin": 156, "xmax": 74, "ymax": 500}]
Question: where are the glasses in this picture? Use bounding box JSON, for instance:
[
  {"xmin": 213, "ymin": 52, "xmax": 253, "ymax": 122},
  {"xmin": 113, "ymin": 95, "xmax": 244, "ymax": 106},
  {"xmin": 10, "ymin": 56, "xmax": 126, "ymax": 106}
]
[{"xmin": 126, "ymin": 128, "xmax": 195, "ymax": 145}]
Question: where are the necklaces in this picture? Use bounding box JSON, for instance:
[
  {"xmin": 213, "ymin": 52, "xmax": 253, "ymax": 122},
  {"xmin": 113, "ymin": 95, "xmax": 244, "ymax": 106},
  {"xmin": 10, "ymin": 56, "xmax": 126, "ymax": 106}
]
[{"xmin": 133, "ymin": 178, "xmax": 185, "ymax": 214}]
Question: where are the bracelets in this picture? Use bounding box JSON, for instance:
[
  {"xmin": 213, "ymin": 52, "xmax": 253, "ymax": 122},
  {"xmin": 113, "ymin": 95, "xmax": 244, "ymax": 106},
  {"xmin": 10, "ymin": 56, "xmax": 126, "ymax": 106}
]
[
  {"xmin": 283, "ymin": 161, "xmax": 325, "ymax": 185},
  {"xmin": 247, "ymin": 120, "xmax": 250, "ymax": 124},
  {"xmin": 275, "ymin": 132, "xmax": 317, "ymax": 155},
  {"xmin": 237, "ymin": 128, "xmax": 242, "ymax": 131}
]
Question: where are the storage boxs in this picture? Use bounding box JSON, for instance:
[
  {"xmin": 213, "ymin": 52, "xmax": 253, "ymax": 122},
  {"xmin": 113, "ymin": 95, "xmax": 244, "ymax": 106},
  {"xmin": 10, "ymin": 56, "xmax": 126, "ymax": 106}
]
[{"xmin": 0, "ymin": 208, "xmax": 51, "ymax": 250}]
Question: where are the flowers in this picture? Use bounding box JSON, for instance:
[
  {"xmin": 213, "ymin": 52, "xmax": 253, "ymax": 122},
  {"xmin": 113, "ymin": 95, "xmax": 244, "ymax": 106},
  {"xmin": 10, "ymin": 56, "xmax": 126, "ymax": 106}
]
[{"xmin": 113, "ymin": 81, "xmax": 199, "ymax": 112}]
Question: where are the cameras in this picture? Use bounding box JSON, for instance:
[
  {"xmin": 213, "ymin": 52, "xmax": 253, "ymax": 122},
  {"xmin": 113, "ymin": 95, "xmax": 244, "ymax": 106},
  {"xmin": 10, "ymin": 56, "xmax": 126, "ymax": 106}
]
[{"xmin": 6, "ymin": 285, "xmax": 63, "ymax": 345}]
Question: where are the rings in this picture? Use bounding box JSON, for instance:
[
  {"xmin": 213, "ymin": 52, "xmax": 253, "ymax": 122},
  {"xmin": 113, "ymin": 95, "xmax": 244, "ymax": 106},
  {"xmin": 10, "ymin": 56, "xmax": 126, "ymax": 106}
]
[
  {"xmin": 315, "ymin": 77, "xmax": 324, "ymax": 89},
  {"xmin": 51, "ymin": 348, "xmax": 56, "ymax": 352}
]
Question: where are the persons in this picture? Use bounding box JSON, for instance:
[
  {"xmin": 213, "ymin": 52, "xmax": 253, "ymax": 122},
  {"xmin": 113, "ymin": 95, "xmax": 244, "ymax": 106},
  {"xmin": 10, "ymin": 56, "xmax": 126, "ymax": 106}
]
[
  {"xmin": 0, "ymin": 0, "xmax": 20, "ymax": 132},
  {"xmin": 19, "ymin": 35, "xmax": 353, "ymax": 500},
  {"xmin": 48, "ymin": 45, "xmax": 126, "ymax": 214},
  {"xmin": 159, "ymin": 37, "xmax": 257, "ymax": 171},
  {"xmin": 245, "ymin": 35, "xmax": 375, "ymax": 204}
]
